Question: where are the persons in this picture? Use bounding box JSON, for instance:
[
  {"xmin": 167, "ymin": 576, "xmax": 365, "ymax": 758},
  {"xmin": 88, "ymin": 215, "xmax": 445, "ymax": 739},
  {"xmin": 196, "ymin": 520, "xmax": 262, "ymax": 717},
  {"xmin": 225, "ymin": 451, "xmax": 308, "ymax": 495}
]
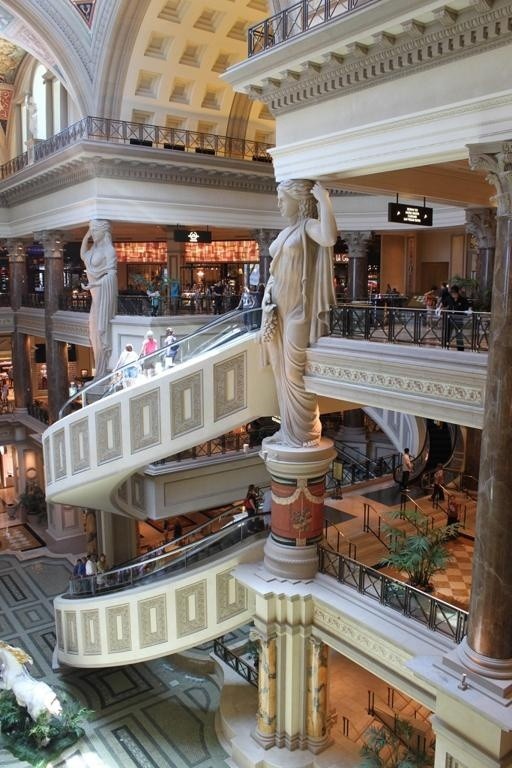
[
  {"xmin": 245, "ymin": 485, "xmax": 273, "ymax": 534},
  {"xmin": 1, "ymin": 380, "xmax": 9, "ymax": 403},
  {"xmin": 446, "ymin": 495, "xmax": 459, "ymax": 540},
  {"xmin": 260, "ymin": 179, "xmax": 339, "ymax": 449},
  {"xmin": 165, "ymin": 328, "xmax": 180, "ymax": 362},
  {"xmin": 174, "ymin": 518, "xmax": 182, "ymax": 546},
  {"xmin": 400, "ymin": 448, "xmax": 411, "ymax": 492},
  {"xmin": 428, "ymin": 464, "xmax": 445, "ymax": 501},
  {"xmin": 423, "ymin": 282, "xmax": 473, "ymax": 352},
  {"xmin": 147, "ymin": 289, "xmax": 161, "ymax": 316},
  {"xmin": 194, "ymin": 281, "xmax": 264, "ymax": 332},
  {"xmin": 140, "ymin": 331, "xmax": 158, "ymax": 376},
  {"xmin": 112, "ymin": 344, "xmax": 140, "ymax": 387},
  {"xmin": 73, "ymin": 553, "xmax": 111, "ymax": 595},
  {"xmin": 386, "ymin": 284, "xmax": 400, "ymax": 296},
  {"xmin": 79, "ymin": 220, "xmax": 119, "ymax": 383}
]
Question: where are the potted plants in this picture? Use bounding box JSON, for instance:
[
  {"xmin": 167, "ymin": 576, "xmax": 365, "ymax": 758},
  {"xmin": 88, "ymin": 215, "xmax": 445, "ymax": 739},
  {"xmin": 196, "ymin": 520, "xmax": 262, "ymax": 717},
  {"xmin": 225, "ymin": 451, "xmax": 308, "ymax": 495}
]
[
  {"xmin": 17, "ymin": 482, "xmax": 50, "ymax": 527},
  {"xmin": 378, "ymin": 502, "xmax": 473, "ymax": 615}
]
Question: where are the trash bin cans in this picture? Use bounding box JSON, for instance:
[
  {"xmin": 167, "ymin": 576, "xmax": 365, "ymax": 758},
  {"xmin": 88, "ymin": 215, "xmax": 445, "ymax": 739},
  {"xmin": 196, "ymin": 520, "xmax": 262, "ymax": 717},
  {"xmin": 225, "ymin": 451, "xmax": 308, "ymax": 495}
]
[{"xmin": 6, "ymin": 504, "xmax": 16, "ymax": 520}]
